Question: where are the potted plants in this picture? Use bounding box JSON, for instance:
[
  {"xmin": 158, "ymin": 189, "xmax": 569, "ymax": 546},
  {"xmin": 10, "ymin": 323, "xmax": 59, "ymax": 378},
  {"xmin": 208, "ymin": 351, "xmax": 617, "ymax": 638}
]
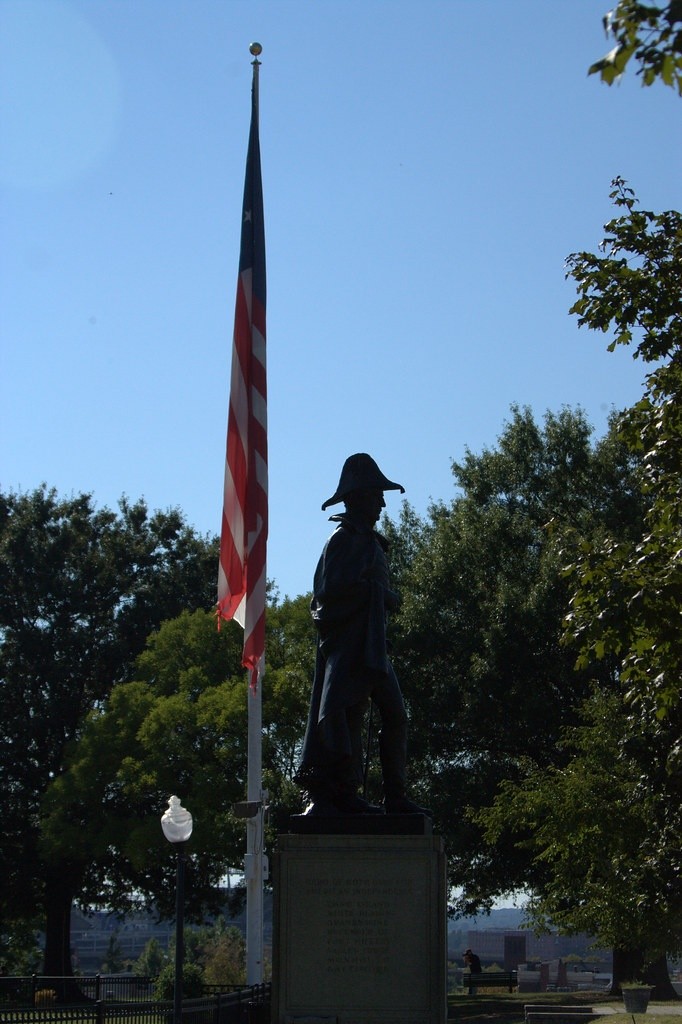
[{"xmin": 621, "ymin": 981, "xmax": 652, "ymax": 1013}]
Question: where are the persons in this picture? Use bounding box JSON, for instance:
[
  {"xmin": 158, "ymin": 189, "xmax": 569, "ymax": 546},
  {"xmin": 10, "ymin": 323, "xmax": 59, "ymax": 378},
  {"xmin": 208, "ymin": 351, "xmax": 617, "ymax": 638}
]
[
  {"xmin": 462, "ymin": 949, "xmax": 482, "ymax": 994},
  {"xmin": 292, "ymin": 451, "xmax": 434, "ymax": 817}
]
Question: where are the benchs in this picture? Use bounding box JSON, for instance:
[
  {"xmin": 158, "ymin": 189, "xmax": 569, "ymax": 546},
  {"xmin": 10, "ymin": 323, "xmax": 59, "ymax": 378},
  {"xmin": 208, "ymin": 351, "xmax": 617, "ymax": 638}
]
[{"xmin": 463, "ymin": 972, "xmax": 519, "ymax": 995}]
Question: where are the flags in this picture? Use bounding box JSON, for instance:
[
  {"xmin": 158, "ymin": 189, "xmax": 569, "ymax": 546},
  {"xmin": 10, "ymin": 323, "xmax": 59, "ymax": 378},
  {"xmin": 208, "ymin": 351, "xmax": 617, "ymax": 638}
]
[{"xmin": 210, "ymin": 83, "xmax": 268, "ymax": 688}]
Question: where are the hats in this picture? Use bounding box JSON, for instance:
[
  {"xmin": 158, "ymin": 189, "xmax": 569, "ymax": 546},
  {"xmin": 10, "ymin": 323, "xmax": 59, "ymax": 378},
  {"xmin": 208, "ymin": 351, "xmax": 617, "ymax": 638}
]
[{"xmin": 465, "ymin": 948, "xmax": 473, "ymax": 955}]
